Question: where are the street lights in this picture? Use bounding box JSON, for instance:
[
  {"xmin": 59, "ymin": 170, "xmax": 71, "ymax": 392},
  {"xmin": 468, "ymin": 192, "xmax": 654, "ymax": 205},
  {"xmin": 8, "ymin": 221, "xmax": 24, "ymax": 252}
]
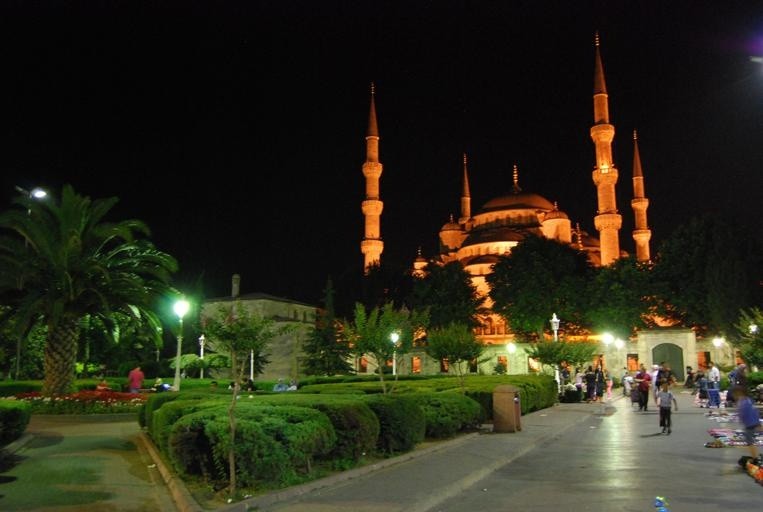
[
  {"xmin": 549, "ymin": 312, "xmax": 561, "ymax": 393},
  {"xmin": 713, "ymin": 337, "xmax": 725, "ymax": 367},
  {"xmin": 172, "ymin": 295, "xmax": 191, "ymax": 391},
  {"xmin": 601, "ymin": 332, "xmax": 624, "ymax": 384},
  {"xmin": 749, "ymin": 323, "xmax": 758, "ymax": 373},
  {"xmin": 390, "ymin": 332, "xmax": 400, "ymax": 375},
  {"xmin": 15, "ymin": 184, "xmax": 49, "ymax": 375},
  {"xmin": 198, "ymin": 334, "xmax": 206, "ymax": 380},
  {"xmin": 507, "ymin": 343, "xmax": 516, "ymax": 375}
]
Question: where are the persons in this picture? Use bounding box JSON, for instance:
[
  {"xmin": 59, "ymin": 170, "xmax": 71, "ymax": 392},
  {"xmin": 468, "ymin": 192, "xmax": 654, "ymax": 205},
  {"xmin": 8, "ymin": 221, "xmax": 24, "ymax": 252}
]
[
  {"xmin": 286, "ymin": 378, "xmax": 297, "ymax": 391},
  {"xmin": 127, "ymin": 364, "xmax": 145, "ymax": 393},
  {"xmin": 272, "ymin": 378, "xmax": 288, "ymax": 391},
  {"xmin": 242, "ymin": 377, "xmax": 256, "ymax": 391},
  {"xmin": 148, "ymin": 377, "xmax": 163, "ymax": 392},
  {"xmin": 557, "ymin": 359, "xmax": 719, "ymax": 435},
  {"xmin": 227, "ymin": 381, "xmax": 241, "ymax": 390},
  {"xmin": 733, "ymin": 391, "xmax": 762, "ymax": 458},
  {"xmin": 95, "ymin": 379, "xmax": 111, "ymax": 391},
  {"xmin": 209, "ymin": 380, "xmax": 217, "ymax": 388},
  {"xmin": 733, "ymin": 354, "xmax": 746, "ymax": 389}
]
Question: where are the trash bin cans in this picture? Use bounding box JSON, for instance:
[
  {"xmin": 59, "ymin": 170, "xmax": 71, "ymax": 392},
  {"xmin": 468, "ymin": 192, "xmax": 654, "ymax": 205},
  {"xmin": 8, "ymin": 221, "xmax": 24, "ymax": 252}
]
[{"xmin": 492, "ymin": 384, "xmax": 521, "ymax": 434}]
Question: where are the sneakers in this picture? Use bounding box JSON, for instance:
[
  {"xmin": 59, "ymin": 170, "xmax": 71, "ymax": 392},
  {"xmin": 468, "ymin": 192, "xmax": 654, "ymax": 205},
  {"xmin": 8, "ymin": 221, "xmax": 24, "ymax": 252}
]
[{"xmin": 661, "ymin": 427, "xmax": 673, "ymax": 436}]
